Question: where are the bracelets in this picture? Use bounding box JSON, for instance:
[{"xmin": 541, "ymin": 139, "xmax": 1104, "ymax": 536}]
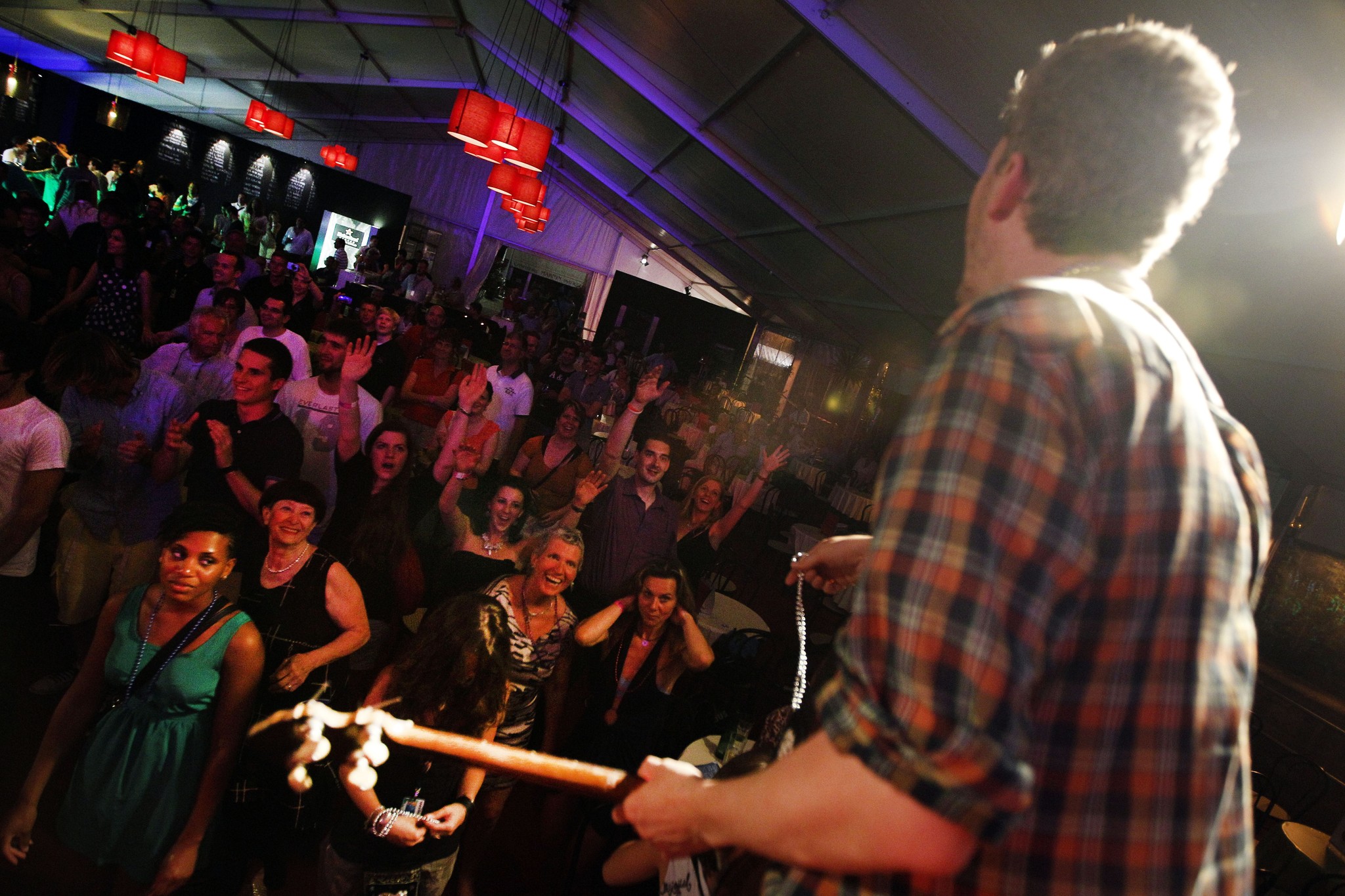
[
  {"xmin": 628, "ymin": 403, "xmax": 644, "ymax": 415},
  {"xmin": 454, "ymin": 796, "xmax": 475, "ymax": 815},
  {"xmin": 613, "ymin": 600, "xmax": 625, "ymax": 613},
  {"xmin": 757, "ymin": 474, "xmax": 766, "ymax": 482},
  {"xmin": 572, "ymin": 504, "xmax": 584, "ymax": 512},
  {"xmin": 338, "ymin": 401, "xmax": 359, "ymax": 408},
  {"xmin": 307, "ymin": 279, "xmax": 312, "ymax": 284},
  {"xmin": 453, "ymin": 470, "xmax": 472, "ymax": 480},
  {"xmin": 366, "ymin": 806, "xmax": 390, "ymax": 830},
  {"xmin": 220, "ymin": 463, "xmax": 238, "ymax": 474},
  {"xmin": 373, "ymin": 808, "xmax": 439, "ymax": 838},
  {"xmin": 458, "ymin": 406, "xmax": 471, "ymax": 416}
]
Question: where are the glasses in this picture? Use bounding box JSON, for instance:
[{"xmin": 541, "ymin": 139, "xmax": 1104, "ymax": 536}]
[{"xmin": 502, "ymin": 342, "xmax": 525, "ymax": 352}]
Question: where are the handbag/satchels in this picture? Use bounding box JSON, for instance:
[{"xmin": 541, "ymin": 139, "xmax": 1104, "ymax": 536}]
[{"xmin": 394, "ymin": 537, "xmax": 424, "ymax": 618}]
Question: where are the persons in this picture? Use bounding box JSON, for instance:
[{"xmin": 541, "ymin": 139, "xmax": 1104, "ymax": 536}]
[
  {"xmin": 0, "ymin": 136, "xmax": 792, "ymax": 896},
  {"xmin": 612, "ymin": 20, "xmax": 1272, "ymax": 896}
]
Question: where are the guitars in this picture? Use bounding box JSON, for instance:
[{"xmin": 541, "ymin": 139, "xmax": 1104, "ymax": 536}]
[{"xmin": 245, "ymin": 701, "xmax": 785, "ymax": 896}]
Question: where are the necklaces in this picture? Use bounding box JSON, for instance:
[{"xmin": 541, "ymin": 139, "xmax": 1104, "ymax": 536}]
[
  {"xmin": 636, "ymin": 626, "xmax": 661, "ymax": 646},
  {"xmin": 790, "ymin": 550, "xmax": 809, "ymax": 711},
  {"xmin": 434, "ymin": 697, "xmax": 446, "ymax": 712},
  {"xmin": 121, "ymin": 588, "xmax": 219, "ymax": 717},
  {"xmin": 523, "ymin": 591, "xmax": 553, "ymax": 616},
  {"xmin": 264, "ymin": 543, "xmax": 309, "ymax": 573},
  {"xmin": 521, "ymin": 577, "xmax": 562, "ymax": 670},
  {"xmin": 481, "ymin": 533, "xmax": 504, "ymax": 554},
  {"xmin": 615, "ymin": 627, "xmax": 672, "ymax": 693}
]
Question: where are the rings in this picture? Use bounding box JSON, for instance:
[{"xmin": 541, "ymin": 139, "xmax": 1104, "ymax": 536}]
[
  {"xmin": 777, "ymin": 456, "xmax": 780, "ymax": 458},
  {"xmin": 288, "ymin": 685, "xmax": 291, "ymax": 689}
]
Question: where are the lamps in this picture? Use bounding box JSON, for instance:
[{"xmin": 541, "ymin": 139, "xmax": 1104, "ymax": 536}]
[
  {"xmin": 685, "ymin": 286, "xmax": 692, "ymax": 297},
  {"xmin": 446, "ymin": 1, "xmax": 577, "ymax": 233},
  {"xmin": 244, "ymin": 1, "xmax": 304, "ymax": 140},
  {"xmin": 641, "ymin": 253, "xmax": 650, "ymax": 266},
  {"xmin": 320, "ymin": 52, "xmax": 367, "ymax": 171},
  {"xmin": 107, "ymin": 1, "xmax": 187, "ymax": 85}
]
